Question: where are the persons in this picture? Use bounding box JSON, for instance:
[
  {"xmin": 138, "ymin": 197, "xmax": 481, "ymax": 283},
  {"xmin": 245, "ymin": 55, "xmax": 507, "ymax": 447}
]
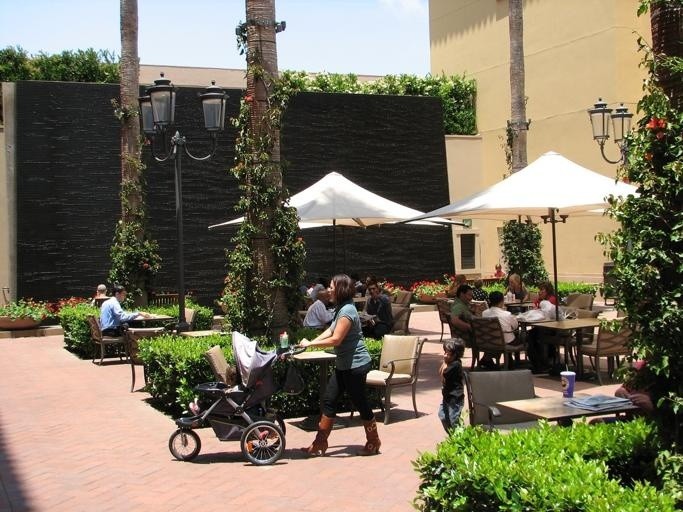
[
  {"xmin": 589, "ymin": 358, "xmax": 667, "ymax": 424},
  {"xmin": 300, "ymin": 272, "xmax": 382, "ymax": 457},
  {"xmin": 188, "ymin": 366, "xmax": 237, "ymax": 417},
  {"xmin": 444, "ymin": 263, "xmax": 563, "ymax": 375},
  {"xmin": 300, "ymin": 273, "xmax": 394, "ymax": 339},
  {"xmin": 437, "ymin": 338, "xmax": 466, "ymax": 434},
  {"xmin": 100, "ymin": 286, "xmax": 150, "ymax": 336},
  {"xmin": 90, "ymin": 283, "xmax": 109, "ymax": 308}
]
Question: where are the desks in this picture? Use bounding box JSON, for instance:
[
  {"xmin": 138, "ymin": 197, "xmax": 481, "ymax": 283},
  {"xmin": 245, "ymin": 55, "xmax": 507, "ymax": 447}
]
[
  {"xmin": 133, "ymin": 313, "xmax": 174, "ymax": 328},
  {"xmin": 496, "ymin": 391, "xmax": 635, "ymax": 438},
  {"xmin": 180, "ymin": 330, "xmax": 233, "ymax": 338},
  {"xmin": 286, "ymin": 347, "xmax": 345, "ymax": 434}
]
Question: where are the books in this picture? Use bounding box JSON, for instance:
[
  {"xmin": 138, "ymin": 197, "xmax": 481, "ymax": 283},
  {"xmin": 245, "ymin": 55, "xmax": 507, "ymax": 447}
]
[{"xmin": 563, "ymin": 393, "xmax": 633, "ymax": 413}]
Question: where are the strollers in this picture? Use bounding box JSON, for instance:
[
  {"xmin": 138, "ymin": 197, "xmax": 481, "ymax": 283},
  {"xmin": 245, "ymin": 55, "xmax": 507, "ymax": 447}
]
[{"xmin": 168, "ymin": 331, "xmax": 307, "ymax": 465}]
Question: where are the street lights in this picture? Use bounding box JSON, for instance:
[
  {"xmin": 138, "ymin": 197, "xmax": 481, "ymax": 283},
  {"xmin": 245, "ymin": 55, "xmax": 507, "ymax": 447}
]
[
  {"xmin": 138, "ymin": 72, "xmax": 230, "ymax": 331},
  {"xmin": 588, "ymin": 98, "xmax": 642, "ymax": 252}
]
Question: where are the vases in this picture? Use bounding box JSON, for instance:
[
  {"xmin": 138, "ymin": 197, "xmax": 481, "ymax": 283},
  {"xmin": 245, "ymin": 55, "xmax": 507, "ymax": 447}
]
[
  {"xmin": 0, "ymin": 315, "xmax": 41, "ymax": 329},
  {"xmin": 40, "ymin": 316, "xmax": 60, "ymax": 325}
]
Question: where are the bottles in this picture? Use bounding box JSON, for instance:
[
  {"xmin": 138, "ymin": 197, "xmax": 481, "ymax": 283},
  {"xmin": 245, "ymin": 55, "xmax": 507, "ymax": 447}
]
[
  {"xmin": 257, "ymin": 408, "xmax": 263, "ymax": 421},
  {"xmin": 365, "ymin": 288, "xmax": 369, "ymax": 300},
  {"xmin": 280, "ymin": 332, "xmax": 288, "ymax": 348}
]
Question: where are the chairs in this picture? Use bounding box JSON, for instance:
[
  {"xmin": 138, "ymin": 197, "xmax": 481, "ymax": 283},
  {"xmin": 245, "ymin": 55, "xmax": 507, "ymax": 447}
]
[
  {"xmin": 166, "ymin": 307, "xmax": 201, "ymax": 334},
  {"xmin": 460, "ymin": 368, "xmax": 546, "ymax": 438},
  {"xmin": 433, "ymin": 287, "xmax": 635, "ymax": 387},
  {"xmin": 95, "ymin": 297, "xmax": 111, "ymax": 308},
  {"xmin": 199, "ymin": 344, "xmax": 230, "ymax": 383},
  {"xmin": 87, "ymin": 315, "xmax": 129, "ymax": 365},
  {"xmin": 123, "ymin": 329, "xmax": 166, "ymax": 392},
  {"xmin": 300, "ymin": 287, "xmax": 417, "ymax": 336},
  {"xmin": 348, "ymin": 332, "xmax": 429, "ymax": 425}
]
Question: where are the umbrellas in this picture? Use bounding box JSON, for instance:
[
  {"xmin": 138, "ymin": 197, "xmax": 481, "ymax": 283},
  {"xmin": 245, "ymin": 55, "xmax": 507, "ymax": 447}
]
[
  {"xmin": 208, "ymin": 170, "xmax": 470, "ymax": 275},
  {"xmin": 387, "ymin": 149, "xmax": 656, "ymax": 322}
]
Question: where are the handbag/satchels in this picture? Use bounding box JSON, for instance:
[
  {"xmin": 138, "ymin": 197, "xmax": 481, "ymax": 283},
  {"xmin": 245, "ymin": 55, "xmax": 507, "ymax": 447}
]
[{"xmin": 270, "ymin": 353, "xmax": 315, "ymax": 395}]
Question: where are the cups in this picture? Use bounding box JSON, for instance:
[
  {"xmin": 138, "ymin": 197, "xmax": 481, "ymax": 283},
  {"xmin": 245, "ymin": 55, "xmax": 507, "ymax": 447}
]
[
  {"xmin": 503, "ymin": 291, "xmax": 515, "ymax": 302},
  {"xmin": 559, "ymin": 371, "xmax": 575, "ymax": 398},
  {"xmin": 356, "ymin": 293, "xmax": 361, "ymax": 300}
]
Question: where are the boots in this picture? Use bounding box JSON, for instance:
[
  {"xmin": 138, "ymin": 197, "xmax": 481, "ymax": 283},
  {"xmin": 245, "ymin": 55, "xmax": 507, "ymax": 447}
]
[
  {"xmin": 301, "ymin": 414, "xmax": 337, "ymax": 457},
  {"xmin": 356, "ymin": 415, "xmax": 381, "ymax": 456}
]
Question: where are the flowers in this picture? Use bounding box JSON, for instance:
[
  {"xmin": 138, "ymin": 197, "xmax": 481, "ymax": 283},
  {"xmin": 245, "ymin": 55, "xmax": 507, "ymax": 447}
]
[{"xmin": 39, "ymin": 296, "xmax": 89, "ymax": 321}]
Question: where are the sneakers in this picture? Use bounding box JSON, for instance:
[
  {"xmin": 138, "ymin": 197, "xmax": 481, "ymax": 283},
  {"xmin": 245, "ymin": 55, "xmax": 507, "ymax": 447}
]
[
  {"xmin": 189, "ymin": 403, "xmax": 198, "ymax": 416},
  {"xmin": 193, "ymin": 398, "xmax": 199, "ymax": 404}
]
